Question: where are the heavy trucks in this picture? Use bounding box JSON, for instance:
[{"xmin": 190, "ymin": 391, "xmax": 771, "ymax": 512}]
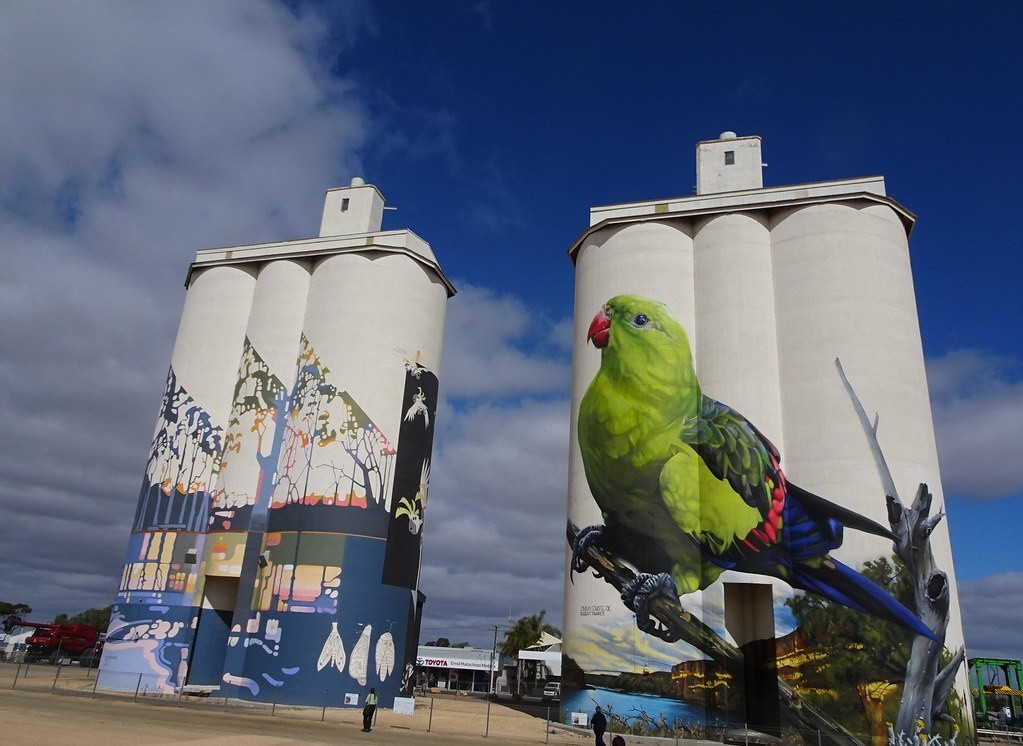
[{"xmin": 12, "ymin": 622, "xmax": 100, "ymax": 666}]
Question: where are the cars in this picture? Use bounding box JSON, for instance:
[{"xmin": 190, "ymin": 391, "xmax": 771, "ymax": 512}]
[{"xmin": 544, "ymin": 682, "xmax": 561, "ymax": 696}]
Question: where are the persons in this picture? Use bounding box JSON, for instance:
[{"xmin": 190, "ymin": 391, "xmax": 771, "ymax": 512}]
[
  {"xmin": 612, "ymin": 735, "xmax": 626, "ymax": 746},
  {"xmin": 997, "ymin": 708, "xmax": 1007, "ymax": 731},
  {"xmin": 413, "ymin": 677, "xmax": 416, "ymax": 695},
  {"xmin": 361, "ymin": 688, "xmax": 377, "ymax": 732},
  {"xmin": 590, "ymin": 706, "xmax": 607, "ymax": 746},
  {"xmin": 417, "ymin": 672, "xmax": 434, "ymax": 697}
]
